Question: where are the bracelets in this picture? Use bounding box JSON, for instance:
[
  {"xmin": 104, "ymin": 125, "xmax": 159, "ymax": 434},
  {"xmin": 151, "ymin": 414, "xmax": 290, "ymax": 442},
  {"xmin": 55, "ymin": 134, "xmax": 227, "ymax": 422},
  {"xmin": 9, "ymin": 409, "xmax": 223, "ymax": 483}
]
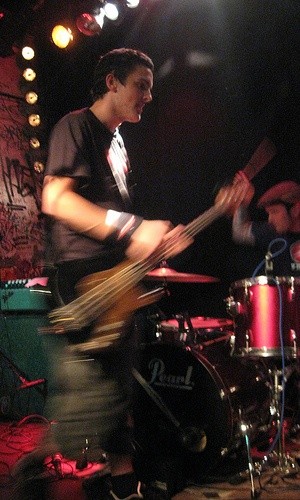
[{"xmin": 107, "ymin": 211, "xmax": 143, "ymax": 250}]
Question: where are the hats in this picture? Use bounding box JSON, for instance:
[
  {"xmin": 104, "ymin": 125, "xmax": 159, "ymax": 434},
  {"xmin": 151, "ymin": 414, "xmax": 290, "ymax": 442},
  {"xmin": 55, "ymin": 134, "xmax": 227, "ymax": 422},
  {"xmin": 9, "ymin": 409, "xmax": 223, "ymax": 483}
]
[{"xmin": 255, "ymin": 179, "xmax": 300, "ymax": 210}]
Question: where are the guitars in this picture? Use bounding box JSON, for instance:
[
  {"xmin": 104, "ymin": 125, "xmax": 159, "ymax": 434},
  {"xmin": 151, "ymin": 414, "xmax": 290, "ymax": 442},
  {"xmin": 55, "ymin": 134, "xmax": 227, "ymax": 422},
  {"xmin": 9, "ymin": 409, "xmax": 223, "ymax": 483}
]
[{"xmin": 47, "ymin": 185, "xmax": 248, "ymax": 360}]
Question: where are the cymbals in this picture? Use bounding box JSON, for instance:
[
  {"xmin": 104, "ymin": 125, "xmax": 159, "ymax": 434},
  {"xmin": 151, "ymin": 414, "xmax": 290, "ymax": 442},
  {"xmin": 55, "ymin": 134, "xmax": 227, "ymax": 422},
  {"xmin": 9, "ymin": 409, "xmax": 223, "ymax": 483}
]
[{"xmin": 144, "ymin": 268, "xmax": 220, "ymax": 283}]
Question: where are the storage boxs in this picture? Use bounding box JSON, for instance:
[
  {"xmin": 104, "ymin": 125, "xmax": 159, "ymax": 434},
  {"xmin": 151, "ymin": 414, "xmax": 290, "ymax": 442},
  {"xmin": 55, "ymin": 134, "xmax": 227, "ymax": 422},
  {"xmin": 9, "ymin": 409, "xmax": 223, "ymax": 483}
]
[{"xmin": 0, "ymin": 288, "xmax": 90, "ymax": 421}]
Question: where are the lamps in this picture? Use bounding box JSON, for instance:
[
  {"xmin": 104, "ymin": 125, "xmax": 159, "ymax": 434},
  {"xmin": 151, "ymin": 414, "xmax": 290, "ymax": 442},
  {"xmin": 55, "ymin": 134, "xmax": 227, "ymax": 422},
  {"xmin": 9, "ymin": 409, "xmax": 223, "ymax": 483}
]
[{"xmin": 51, "ymin": 0, "xmax": 140, "ymax": 49}]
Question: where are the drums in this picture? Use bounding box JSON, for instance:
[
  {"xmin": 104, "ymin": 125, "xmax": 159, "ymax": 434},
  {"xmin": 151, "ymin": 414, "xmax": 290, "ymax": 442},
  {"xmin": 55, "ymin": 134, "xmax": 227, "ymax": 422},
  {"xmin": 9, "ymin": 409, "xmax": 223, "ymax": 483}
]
[
  {"xmin": 126, "ymin": 333, "xmax": 276, "ymax": 482},
  {"xmin": 158, "ymin": 316, "xmax": 233, "ymax": 345},
  {"xmin": 230, "ymin": 276, "xmax": 300, "ymax": 359}
]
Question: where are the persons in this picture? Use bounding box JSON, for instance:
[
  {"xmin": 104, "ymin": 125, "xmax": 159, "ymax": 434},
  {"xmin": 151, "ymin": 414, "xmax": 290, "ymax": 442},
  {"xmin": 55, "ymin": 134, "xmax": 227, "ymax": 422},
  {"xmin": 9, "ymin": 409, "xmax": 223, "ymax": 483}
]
[
  {"xmin": 40, "ymin": 47, "xmax": 195, "ymax": 500},
  {"xmin": 229, "ymin": 169, "xmax": 300, "ymax": 437}
]
